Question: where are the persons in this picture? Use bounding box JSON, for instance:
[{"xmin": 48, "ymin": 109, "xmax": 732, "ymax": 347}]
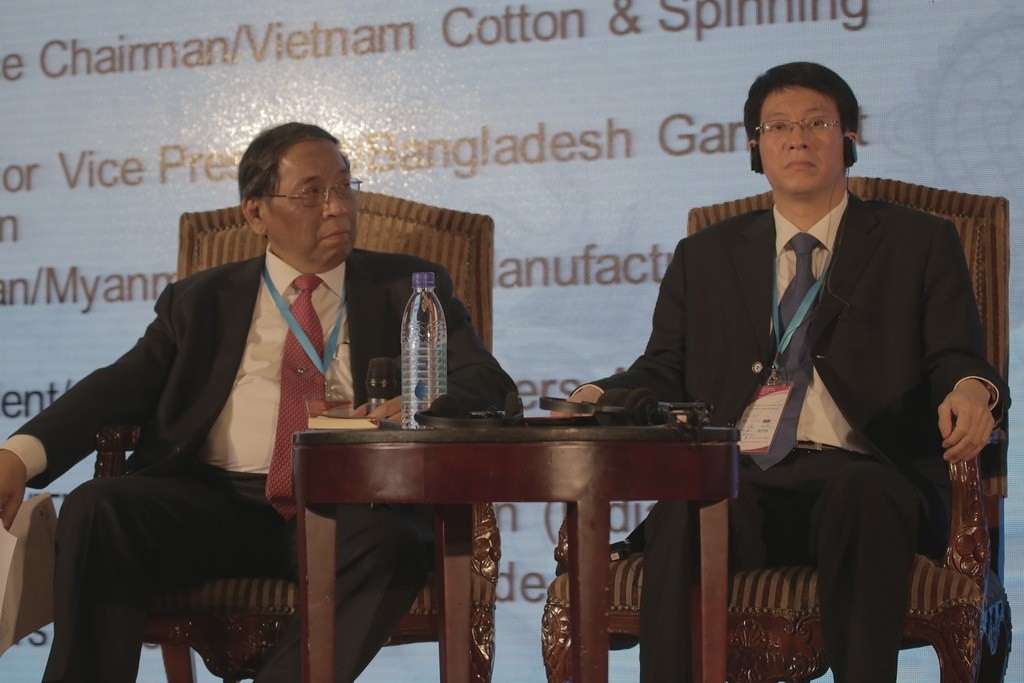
[
  {"xmin": 547, "ymin": 61, "xmax": 1013, "ymax": 683},
  {"xmin": 0, "ymin": 124, "xmax": 521, "ymax": 683}
]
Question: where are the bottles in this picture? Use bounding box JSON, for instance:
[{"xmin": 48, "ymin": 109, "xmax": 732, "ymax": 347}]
[{"xmin": 401, "ymin": 271, "xmax": 447, "ymax": 430}]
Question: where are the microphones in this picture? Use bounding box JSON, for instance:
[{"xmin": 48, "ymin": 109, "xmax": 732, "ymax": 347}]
[{"xmin": 366, "ymin": 358, "xmax": 398, "ymax": 414}]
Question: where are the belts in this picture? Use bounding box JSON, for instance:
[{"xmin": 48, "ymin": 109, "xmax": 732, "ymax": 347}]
[{"xmin": 791, "ymin": 440, "xmax": 872, "ymax": 457}]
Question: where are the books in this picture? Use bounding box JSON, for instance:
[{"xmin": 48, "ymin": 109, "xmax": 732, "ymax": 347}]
[{"xmin": 307, "ymin": 415, "xmax": 400, "ymax": 430}]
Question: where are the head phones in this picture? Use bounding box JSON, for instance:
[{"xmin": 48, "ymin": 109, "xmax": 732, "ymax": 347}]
[
  {"xmin": 413, "ymin": 391, "xmax": 524, "ymax": 430},
  {"xmin": 539, "ymin": 383, "xmax": 664, "ymax": 426},
  {"xmin": 748, "ymin": 122, "xmax": 858, "ymax": 176}
]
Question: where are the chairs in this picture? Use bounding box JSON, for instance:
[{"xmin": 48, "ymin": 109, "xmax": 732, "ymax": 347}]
[
  {"xmin": 90, "ymin": 187, "xmax": 501, "ymax": 682},
  {"xmin": 542, "ymin": 177, "xmax": 1011, "ymax": 682}
]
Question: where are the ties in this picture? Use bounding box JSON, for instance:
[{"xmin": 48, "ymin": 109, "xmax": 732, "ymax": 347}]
[
  {"xmin": 264, "ymin": 273, "xmax": 324, "ymax": 522},
  {"xmin": 744, "ymin": 231, "xmax": 825, "ymax": 472}
]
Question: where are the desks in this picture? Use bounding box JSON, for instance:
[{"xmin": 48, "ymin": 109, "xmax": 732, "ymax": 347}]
[{"xmin": 291, "ymin": 426, "xmax": 741, "ymax": 683}]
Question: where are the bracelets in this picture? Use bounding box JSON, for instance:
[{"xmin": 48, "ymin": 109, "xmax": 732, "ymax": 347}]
[{"xmin": 982, "ymin": 381, "xmax": 997, "ymax": 403}]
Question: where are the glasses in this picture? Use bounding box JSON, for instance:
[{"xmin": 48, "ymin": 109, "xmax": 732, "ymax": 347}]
[
  {"xmin": 263, "ymin": 175, "xmax": 362, "ymax": 207},
  {"xmin": 754, "ymin": 119, "xmax": 849, "ymax": 137}
]
